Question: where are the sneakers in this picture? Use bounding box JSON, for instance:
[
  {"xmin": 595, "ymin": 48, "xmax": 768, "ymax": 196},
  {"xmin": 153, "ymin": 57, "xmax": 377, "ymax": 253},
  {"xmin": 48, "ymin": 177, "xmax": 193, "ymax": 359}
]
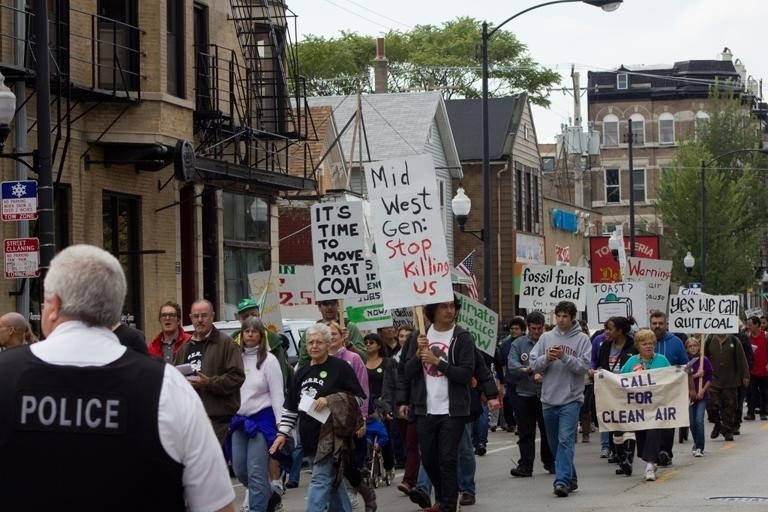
[
  {"xmin": 742, "ymin": 412, "xmax": 768, "ymax": 421},
  {"xmin": 542, "ymin": 461, "xmax": 556, "ymax": 473},
  {"xmin": 421, "ymin": 501, "xmax": 458, "ymax": 512},
  {"xmin": 553, "ymin": 479, "xmax": 579, "ymax": 497},
  {"xmin": 459, "ymin": 493, "xmax": 476, "ymax": 505},
  {"xmin": 692, "ymin": 444, "xmax": 703, "ymax": 457},
  {"xmin": 644, "ymin": 467, "xmax": 655, "ymax": 482},
  {"xmin": 397, "ymin": 481, "xmax": 432, "ymax": 507},
  {"xmin": 601, "ymin": 447, "xmax": 632, "ymax": 477},
  {"xmin": 510, "ymin": 464, "xmax": 532, "ymax": 477}
]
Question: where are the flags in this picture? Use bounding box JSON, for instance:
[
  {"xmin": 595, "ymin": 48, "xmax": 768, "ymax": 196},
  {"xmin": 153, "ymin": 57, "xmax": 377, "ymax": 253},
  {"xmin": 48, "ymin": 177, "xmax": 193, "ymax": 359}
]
[{"xmin": 456, "ymin": 251, "xmax": 480, "ymax": 301}]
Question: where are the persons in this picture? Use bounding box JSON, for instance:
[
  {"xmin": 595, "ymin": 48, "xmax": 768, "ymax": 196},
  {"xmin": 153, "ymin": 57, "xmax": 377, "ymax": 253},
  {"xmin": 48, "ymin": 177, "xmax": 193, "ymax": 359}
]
[
  {"xmin": 0, "ymin": 243, "xmax": 235, "ymax": 512},
  {"xmin": 0, "ymin": 312, "xmax": 27, "ymax": 353}
]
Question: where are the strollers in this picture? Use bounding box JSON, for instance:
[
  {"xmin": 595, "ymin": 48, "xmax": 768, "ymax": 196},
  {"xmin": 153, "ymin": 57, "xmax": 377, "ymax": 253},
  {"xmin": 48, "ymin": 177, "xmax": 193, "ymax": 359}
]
[{"xmin": 360, "ymin": 406, "xmax": 397, "ymax": 490}]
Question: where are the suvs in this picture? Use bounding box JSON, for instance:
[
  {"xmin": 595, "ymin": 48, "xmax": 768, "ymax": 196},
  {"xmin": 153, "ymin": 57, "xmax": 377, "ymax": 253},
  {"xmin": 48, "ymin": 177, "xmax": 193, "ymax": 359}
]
[{"xmin": 172, "ymin": 317, "xmax": 323, "ymax": 370}]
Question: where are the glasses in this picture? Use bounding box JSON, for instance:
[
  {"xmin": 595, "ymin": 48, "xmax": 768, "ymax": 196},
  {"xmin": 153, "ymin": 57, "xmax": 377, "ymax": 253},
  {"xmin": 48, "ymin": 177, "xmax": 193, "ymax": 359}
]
[
  {"xmin": 364, "ymin": 340, "xmax": 377, "ymax": 345},
  {"xmin": 161, "ymin": 312, "xmax": 177, "ymax": 319}
]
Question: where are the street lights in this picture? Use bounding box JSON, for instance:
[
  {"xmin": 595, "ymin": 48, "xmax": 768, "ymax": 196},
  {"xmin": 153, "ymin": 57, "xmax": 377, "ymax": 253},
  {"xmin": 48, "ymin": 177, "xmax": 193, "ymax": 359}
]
[
  {"xmin": 0, "ymin": 68, "xmax": 59, "ymax": 333},
  {"xmin": 682, "ymin": 147, "xmax": 767, "ymax": 285},
  {"xmin": 450, "ymin": 0, "xmax": 623, "ymax": 307}
]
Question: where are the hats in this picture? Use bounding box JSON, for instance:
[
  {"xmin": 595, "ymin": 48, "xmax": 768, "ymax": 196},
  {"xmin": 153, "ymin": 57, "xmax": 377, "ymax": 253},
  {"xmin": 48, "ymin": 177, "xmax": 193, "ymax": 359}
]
[{"xmin": 237, "ymin": 299, "xmax": 258, "ymax": 315}]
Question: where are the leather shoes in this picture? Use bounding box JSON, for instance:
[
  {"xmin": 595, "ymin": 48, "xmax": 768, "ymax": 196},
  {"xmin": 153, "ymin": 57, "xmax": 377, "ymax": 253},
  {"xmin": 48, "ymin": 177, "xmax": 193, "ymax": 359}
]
[{"xmin": 711, "ymin": 423, "xmax": 740, "ymax": 441}]
